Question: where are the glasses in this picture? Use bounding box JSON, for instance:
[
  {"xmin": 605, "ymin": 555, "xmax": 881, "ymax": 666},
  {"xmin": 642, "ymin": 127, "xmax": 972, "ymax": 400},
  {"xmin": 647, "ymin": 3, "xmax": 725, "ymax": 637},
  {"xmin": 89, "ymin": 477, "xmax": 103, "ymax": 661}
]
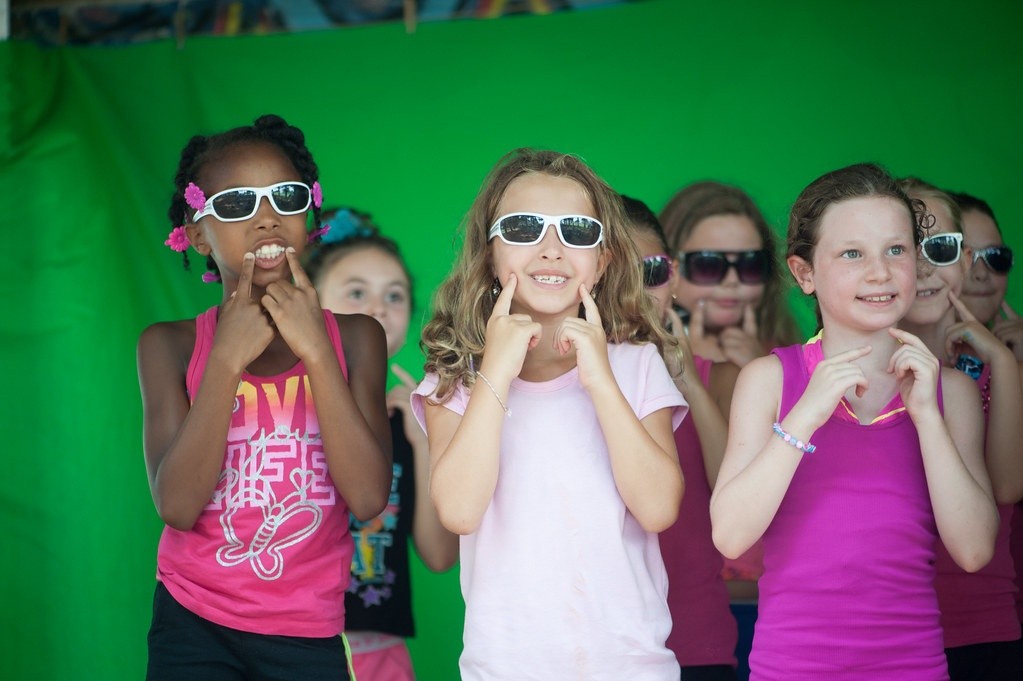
[
  {"xmin": 972, "ymin": 247, "xmax": 1013, "ymax": 273},
  {"xmin": 679, "ymin": 249, "xmax": 773, "ymax": 284},
  {"xmin": 640, "ymin": 255, "xmax": 674, "ymax": 289},
  {"xmin": 191, "ymin": 181, "xmax": 312, "ymax": 223},
  {"xmin": 487, "ymin": 212, "xmax": 603, "ymax": 248},
  {"xmin": 920, "ymin": 232, "xmax": 963, "ymax": 265}
]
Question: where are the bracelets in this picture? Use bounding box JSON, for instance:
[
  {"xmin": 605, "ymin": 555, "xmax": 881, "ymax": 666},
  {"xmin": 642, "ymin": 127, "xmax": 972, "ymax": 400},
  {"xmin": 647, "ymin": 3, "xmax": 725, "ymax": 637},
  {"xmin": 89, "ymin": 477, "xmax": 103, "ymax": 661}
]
[
  {"xmin": 475, "ymin": 369, "xmax": 511, "ymax": 416},
  {"xmin": 772, "ymin": 423, "xmax": 816, "ymax": 452}
]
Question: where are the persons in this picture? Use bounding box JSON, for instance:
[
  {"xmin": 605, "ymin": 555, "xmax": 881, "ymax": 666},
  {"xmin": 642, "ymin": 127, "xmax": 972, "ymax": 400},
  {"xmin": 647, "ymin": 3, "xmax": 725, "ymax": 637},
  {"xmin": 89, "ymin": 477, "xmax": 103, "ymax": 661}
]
[{"xmin": 137, "ymin": 115, "xmax": 1023, "ymax": 681}]
